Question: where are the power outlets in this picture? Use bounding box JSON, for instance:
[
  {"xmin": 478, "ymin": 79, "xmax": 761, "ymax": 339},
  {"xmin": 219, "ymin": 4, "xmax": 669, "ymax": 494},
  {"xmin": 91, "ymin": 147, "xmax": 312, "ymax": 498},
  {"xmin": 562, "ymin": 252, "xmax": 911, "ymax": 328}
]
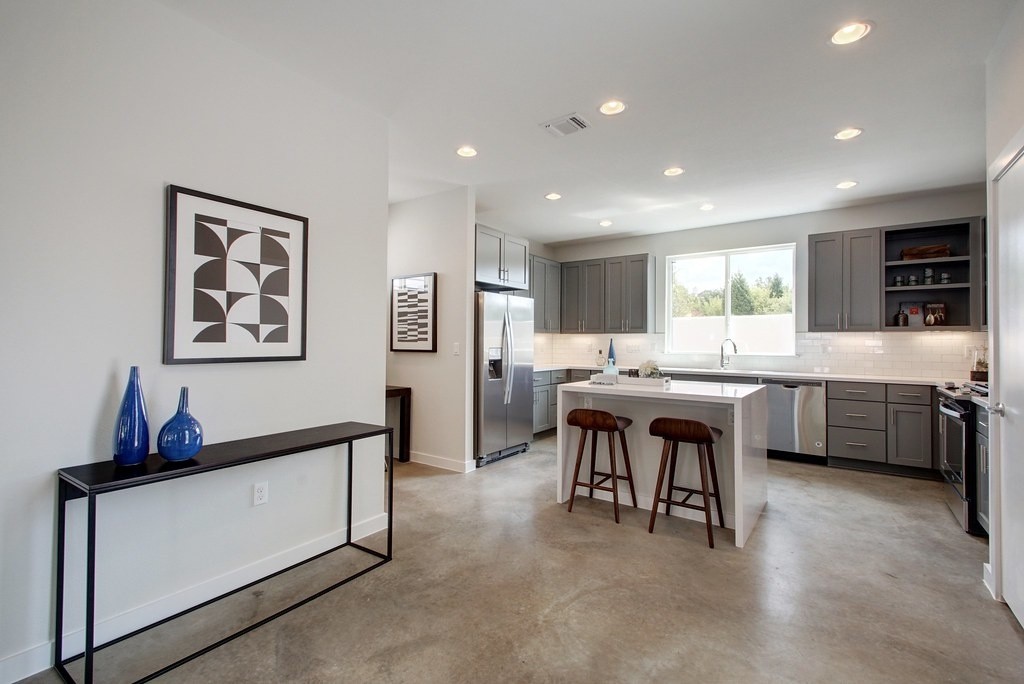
[
  {"xmin": 253, "ymin": 480, "xmax": 268, "ymax": 507},
  {"xmin": 964, "ymin": 345, "xmax": 977, "ymax": 359}
]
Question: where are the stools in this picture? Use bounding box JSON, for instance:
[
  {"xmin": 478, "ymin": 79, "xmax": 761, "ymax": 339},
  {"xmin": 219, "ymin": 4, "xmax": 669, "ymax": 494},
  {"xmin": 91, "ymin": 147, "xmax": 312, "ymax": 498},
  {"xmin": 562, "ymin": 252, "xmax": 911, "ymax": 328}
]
[
  {"xmin": 567, "ymin": 409, "xmax": 638, "ymax": 524},
  {"xmin": 648, "ymin": 415, "xmax": 725, "ymax": 547}
]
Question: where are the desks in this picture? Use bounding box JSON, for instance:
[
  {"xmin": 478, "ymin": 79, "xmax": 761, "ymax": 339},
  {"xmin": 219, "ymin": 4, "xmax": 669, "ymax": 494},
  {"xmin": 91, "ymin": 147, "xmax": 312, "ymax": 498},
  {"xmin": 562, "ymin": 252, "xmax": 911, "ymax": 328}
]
[
  {"xmin": 556, "ymin": 378, "xmax": 768, "ymax": 548},
  {"xmin": 386, "ymin": 385, "xmax": 411, "ymax": 463},
  {"xmin": 54, "ymin": 421, "xmax": 395, "ymax": 684}
]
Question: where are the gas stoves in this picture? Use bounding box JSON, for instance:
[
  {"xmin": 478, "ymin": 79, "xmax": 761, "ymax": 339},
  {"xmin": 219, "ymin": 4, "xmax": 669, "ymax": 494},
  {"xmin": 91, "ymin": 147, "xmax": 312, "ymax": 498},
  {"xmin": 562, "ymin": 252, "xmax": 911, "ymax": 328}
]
[{"xmin": 936, "ymin": 381, "xmax": 988, "ymax": 400}]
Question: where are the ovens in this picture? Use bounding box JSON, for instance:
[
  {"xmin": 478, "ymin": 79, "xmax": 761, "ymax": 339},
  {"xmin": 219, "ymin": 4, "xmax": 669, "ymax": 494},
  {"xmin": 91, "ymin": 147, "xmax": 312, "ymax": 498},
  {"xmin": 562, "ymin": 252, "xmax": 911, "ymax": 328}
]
[{"xmin": 936, "ymin": 398, "xmax": 977, "ymax": 532}]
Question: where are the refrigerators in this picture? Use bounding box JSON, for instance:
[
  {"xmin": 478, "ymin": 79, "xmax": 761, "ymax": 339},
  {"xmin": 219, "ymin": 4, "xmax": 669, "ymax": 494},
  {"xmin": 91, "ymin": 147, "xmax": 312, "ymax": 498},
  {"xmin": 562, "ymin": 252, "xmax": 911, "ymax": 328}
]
[{"xmin": 474, "ymin": 292, "xmax": 534, "ymax": 466}]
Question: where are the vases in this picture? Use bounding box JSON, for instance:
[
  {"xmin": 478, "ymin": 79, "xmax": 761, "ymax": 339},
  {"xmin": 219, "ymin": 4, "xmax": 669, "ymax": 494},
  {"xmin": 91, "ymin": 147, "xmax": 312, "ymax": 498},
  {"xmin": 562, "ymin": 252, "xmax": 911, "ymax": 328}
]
[
  {"xmin": 111, "ymin": 366, "xmax": 150, "ymax": 466},
  {"xmin": 642, "ymin": 371, "xmax": 660, "ymax": 378},
  {"xmin": 158, "ymin": 387, "xmax": 203, "ymax": 463}
]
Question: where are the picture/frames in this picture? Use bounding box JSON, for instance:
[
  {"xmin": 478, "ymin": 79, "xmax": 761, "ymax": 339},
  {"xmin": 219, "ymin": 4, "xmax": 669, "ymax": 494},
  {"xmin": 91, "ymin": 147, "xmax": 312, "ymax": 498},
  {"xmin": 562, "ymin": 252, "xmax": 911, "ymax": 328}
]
[
  {"xmin": 389, "ymin": 272, "xmax": 438, "ymax": 352},
  {"xmin": 163, "ymin": 184, "xmax": 309, "ymax": 365}
]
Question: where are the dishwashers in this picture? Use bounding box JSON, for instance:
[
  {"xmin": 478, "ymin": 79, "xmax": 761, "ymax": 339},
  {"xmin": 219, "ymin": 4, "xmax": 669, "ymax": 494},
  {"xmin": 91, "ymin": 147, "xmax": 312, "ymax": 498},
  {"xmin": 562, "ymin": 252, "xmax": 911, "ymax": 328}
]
[{"xmin": 757, "ymin": 378, "xmax": 827, "ymax": 466}]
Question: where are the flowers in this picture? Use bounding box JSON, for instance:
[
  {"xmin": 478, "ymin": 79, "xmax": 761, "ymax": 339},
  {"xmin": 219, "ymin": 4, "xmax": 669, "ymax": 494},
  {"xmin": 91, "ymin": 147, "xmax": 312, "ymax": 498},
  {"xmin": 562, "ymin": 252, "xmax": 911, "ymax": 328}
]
[{"xmin": 638, "ymin": 360, "xmax": 664, "ymax": 377}]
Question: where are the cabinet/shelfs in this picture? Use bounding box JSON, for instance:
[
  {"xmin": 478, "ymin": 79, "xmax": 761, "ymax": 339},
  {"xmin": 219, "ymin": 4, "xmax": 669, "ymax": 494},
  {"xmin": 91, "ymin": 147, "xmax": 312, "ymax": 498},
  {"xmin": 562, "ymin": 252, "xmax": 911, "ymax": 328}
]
[
  {"xmin": 827, "ymin": 381, "xmax": 887, "ymax": 464},
  {"xmin": 975, "ymin": 404, "xmax": 990, "ymax": 534},
  {"xmin": 886, "ymin": 384, "xmax": 933, "ymax": 470},
  {"xmin": 499, "ymin": 253, "xmax": 657, "ymax": 334},
  {"xmin": 475, "ymin": 223, "xmax": 530, "ymax": 293},
  {"xmin": 807, "ymin": 215, "xmax": 986, "ymax": 332},
  {"xmin": 533, "ymin": 370, "xmax": 628, "ymax": 435}
]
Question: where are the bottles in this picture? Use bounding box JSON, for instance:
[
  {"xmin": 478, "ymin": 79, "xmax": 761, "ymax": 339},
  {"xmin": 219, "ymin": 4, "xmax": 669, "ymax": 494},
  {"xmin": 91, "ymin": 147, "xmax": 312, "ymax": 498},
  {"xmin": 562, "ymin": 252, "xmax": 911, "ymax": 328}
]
[
  {"xmin": 607, "ymin": 338, "xmax": 616, "ymax": 366},
  {"xmin": 157, "ymin": 387, "xmax": 203, "ymax": 463},
  {"xmin": 897, "ymin": 310, "xmax": 908, "ymax": 326},
  {"xmin": 596, "ymin": 350, "xmax": 606, "ymax": 366},
  {"xmin": 112, "ymin": 366, "xmax": 150, "ymax": 466}
]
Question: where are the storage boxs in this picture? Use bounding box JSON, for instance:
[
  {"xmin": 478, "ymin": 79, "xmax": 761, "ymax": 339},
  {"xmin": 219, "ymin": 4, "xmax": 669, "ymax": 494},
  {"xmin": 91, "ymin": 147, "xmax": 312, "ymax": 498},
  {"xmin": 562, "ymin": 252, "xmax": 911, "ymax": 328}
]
[
  {"xmin": 900, "ymin": 302, "xmax": 925, "ymax": 326},
  {"xmin": 618, "ymin": 375, "xmax": 671, "ymax": 386},
  {"xmin": 924, "ymin": 304, "xmax": 946, "ymax": 325}
]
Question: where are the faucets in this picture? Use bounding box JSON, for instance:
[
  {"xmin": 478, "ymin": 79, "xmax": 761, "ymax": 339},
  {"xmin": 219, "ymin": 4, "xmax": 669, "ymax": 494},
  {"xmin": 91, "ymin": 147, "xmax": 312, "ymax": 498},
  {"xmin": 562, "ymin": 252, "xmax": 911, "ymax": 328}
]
[{"xmin": 720, "ymin": 338, "xmax": 738, "ymax": 369}]
